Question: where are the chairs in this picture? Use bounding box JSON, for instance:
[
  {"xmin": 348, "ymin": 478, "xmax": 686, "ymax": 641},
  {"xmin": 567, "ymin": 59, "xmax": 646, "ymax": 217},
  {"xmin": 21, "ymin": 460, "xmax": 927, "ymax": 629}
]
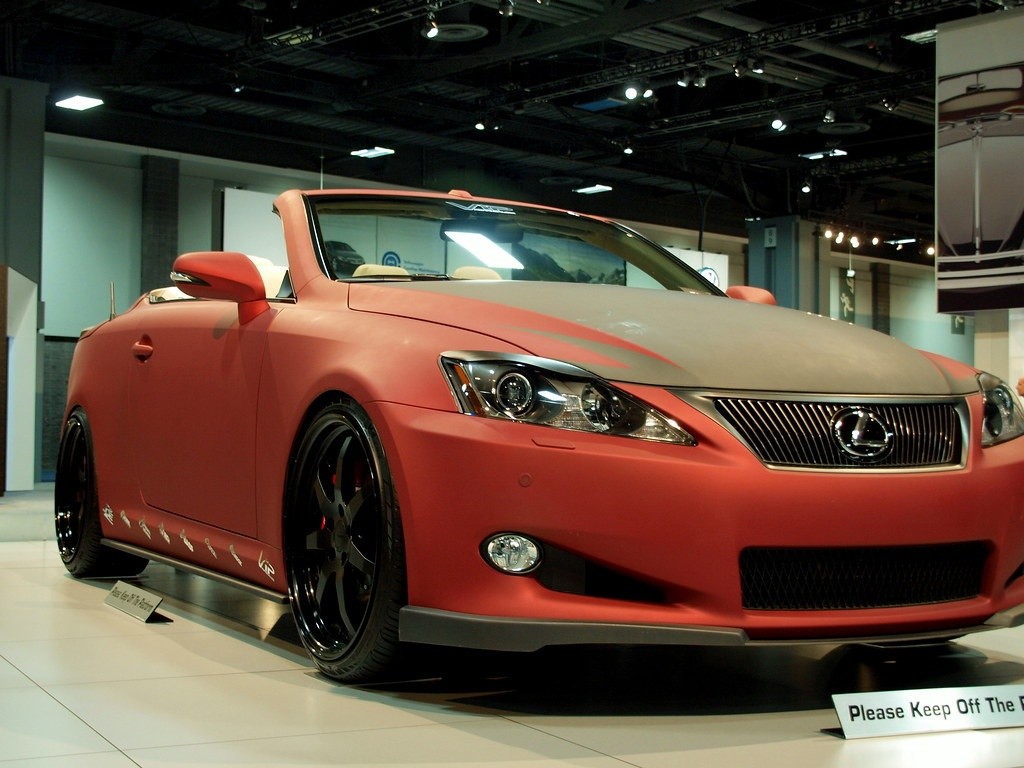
[
  {"xmin": 452, "ymin": 265, "xmax": 504, "ymax": 281},
  {"xmin": 352, "ymin": 264, "xmax": 412, "ymax": 281}
]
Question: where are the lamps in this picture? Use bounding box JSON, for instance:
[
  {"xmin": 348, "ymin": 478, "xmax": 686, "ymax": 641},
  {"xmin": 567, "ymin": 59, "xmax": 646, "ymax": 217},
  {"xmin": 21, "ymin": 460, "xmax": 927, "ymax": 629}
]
[
  {"xmin": 676, "ymin": 68, "xmax": 707, "ymax": 88},
  {"xmin": 733, "ymin": 55, "xmax": 766, "ymax": 79},
  {"xmin": 419, "ymin": 12, "xmax": 439, "ymax": 41},
  {"xmin": 498, "ymin": 0, "xmax": 515, "ymax": 17}
]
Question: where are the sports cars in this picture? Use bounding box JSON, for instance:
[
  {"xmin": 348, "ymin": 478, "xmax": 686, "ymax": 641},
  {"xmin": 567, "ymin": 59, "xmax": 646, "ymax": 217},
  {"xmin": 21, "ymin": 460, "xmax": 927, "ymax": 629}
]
[{"xmin": 49, "ymin": 181, "xmax": 1024, "ymax": 688}]
